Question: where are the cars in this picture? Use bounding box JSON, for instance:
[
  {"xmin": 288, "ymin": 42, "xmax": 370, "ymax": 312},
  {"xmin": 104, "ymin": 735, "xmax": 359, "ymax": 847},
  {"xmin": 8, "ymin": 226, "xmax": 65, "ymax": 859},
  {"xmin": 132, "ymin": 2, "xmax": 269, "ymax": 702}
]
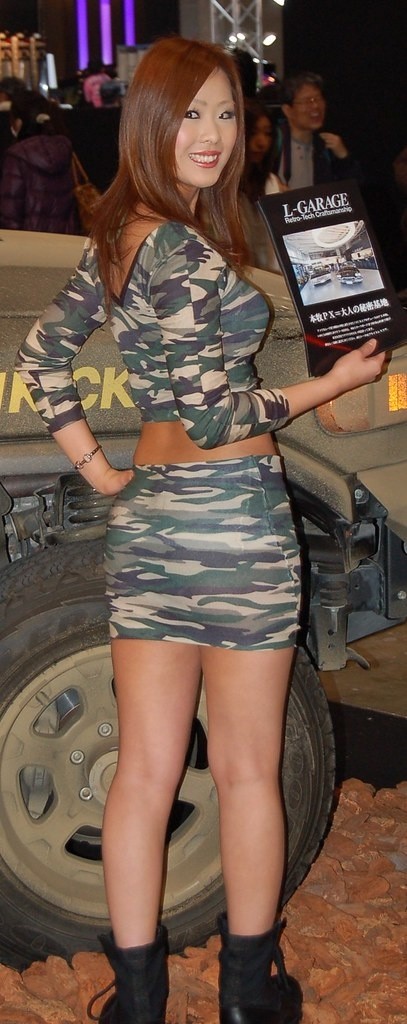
[{"xmin": 335, "ymin": 266, "xmax": 364, "ymax": 286}]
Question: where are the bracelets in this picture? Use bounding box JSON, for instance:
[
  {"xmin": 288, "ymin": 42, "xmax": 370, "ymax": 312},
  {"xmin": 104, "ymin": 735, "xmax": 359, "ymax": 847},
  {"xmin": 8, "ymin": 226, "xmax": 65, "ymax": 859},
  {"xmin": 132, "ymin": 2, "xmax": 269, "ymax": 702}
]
[{"xmin": 72, "ymin": 445, "xmax": 107, "ymax": 470}]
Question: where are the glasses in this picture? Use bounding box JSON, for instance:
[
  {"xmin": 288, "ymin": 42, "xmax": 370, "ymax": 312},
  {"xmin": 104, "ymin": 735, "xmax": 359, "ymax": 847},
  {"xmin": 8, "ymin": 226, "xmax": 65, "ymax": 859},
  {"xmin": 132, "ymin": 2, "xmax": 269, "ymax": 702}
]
[{"xmin": 291, "ymin": 96, "xmax": 322, "ymax": 104}]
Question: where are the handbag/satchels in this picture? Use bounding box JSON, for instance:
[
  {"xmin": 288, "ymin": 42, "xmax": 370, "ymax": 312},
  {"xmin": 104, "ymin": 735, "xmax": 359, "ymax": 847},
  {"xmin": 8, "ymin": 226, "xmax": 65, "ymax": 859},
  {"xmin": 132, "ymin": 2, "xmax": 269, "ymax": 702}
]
[{"xmin": 68, "ymin": 151, "xmax": 103, "ymax": 236}]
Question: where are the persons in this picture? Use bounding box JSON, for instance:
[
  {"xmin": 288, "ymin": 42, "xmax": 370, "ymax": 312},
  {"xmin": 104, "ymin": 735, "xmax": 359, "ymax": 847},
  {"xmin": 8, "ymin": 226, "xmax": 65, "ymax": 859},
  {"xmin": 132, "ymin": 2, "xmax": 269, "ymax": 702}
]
[
  {"xmin": 13, "ymin": 37, "xmax": 385, "ymax": 1024},
  {"xmin": 0, "ymin": 58, "xmax": 407, "ymax": 300}
]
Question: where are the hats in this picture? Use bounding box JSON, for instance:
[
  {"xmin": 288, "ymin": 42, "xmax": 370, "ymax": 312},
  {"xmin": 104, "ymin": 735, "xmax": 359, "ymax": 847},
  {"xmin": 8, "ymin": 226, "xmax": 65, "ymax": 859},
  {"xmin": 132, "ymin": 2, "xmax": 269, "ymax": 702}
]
[{"xmin": 0, "ymin": 75, "xmax": 25, "ymax": 98}]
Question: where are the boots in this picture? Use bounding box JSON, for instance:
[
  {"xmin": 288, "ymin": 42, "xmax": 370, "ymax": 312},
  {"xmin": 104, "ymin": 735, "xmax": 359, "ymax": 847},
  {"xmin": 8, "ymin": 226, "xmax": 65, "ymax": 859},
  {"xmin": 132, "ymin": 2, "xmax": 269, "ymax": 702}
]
[
  {"xmin": 215, "ymin": 912, "xmax": 304, "ymax": 1024},
  {"xmin": 87, "ymin": 925, "xmax": 169, "ymax": 1024}
]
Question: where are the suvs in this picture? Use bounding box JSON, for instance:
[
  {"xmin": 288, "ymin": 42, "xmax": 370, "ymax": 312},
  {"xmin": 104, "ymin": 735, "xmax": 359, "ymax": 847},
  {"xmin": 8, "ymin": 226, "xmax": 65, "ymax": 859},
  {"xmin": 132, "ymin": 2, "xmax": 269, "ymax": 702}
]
[{"xmin": 0, "ymin": 225, "xmax": 407, "ymax": 971}]
